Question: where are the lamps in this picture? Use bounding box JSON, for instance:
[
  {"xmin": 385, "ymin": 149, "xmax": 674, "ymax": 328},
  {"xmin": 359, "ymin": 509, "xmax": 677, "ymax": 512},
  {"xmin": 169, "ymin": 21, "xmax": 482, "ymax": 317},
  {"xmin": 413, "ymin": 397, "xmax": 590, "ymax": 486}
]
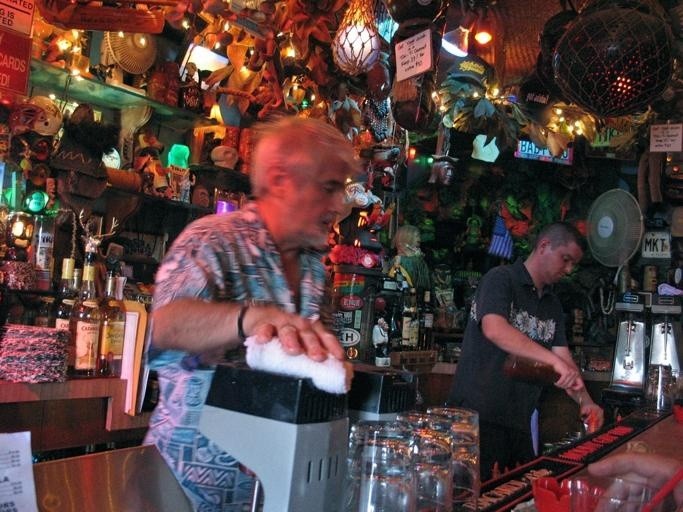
[{"xmin": 442, "ymin": 4, "xmax": 480, "ymax": 58}]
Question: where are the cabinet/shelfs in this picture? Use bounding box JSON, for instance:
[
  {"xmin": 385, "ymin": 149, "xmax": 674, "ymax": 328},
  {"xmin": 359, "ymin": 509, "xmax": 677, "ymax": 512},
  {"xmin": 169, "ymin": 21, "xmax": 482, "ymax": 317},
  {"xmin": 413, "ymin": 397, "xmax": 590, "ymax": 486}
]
[{"xmin": 28, "ymin": 57, "xmax": 249, "ymax": 290}]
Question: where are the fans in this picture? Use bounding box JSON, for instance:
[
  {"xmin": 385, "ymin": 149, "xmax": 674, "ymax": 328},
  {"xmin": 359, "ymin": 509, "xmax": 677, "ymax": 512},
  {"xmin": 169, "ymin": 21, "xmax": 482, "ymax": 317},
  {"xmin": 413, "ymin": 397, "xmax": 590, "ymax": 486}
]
[
  {"xmin": 99, "ymin": 29, "xmax": 158, "ymax": 98},
  {"xmin": 585, "ymin": 188, "xmax": 645, "ymax": 298}
]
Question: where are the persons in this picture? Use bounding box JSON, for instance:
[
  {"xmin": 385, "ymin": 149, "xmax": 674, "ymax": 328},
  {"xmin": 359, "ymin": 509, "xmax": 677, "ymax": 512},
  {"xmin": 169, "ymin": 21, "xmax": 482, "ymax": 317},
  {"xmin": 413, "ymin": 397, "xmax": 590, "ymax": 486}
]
[
  {"xmin": 428, "ymin": 152, "xmax": 460, "ymax": 186},
  {"xmin": 390, "ymin": 222, "xmax": 434, "ymax": 290},
  {"xmin": 122, "ymin": 116, "xmax": 362, "ymax": 512},
  {"xmin": 587, "ymin": 447, "xmax": 683, "ymax": 512},
  {"xmin": 448, "ymin": 219, "xmax": 608, "ymax": 484}
]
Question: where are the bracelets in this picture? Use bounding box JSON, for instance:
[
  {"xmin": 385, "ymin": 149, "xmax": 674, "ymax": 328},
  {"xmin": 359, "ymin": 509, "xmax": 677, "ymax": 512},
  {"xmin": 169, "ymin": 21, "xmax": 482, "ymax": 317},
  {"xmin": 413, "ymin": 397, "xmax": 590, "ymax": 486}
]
[{"xmin": 236, "ymin": 296, "xmax": 282, "ymax": 344}]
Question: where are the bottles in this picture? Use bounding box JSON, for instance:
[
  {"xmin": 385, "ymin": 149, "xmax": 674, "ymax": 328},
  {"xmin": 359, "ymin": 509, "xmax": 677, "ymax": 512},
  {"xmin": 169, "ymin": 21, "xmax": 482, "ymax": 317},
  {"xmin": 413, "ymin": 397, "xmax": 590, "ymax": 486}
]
[
  {"xmin": 504, "ymin": 354, "xmax": 579, "ymax": 390},
  {"xmin": 401, "ymin": 287, "xmax": 434, "ymax": 352},
  {"xmin": 35, "ymin": 251, "xmax": 127, "ymax": 378},
  {"xmin": 137, "ymin": 128, "xmax": 169, "ymax": 192}
]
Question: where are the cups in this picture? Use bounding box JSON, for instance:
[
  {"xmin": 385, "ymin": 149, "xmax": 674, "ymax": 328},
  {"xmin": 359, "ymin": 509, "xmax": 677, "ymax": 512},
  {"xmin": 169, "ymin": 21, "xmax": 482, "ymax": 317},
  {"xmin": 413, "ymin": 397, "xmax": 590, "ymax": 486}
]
[
  {"xmin": 344, "ymin": 407, "xmax": 479, "ymax": 512},
  {"xmin": 571, "ymin": 474, "xmax": 662, "ymax": 512}
]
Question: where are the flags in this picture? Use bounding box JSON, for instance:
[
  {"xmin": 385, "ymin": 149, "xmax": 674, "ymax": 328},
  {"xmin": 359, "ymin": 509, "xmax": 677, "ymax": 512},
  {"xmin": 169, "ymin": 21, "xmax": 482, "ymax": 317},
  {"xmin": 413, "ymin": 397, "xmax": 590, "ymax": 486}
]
[{"xmin": 487, "ymin": 205, "xmax": 515, "ymax": 261}]
